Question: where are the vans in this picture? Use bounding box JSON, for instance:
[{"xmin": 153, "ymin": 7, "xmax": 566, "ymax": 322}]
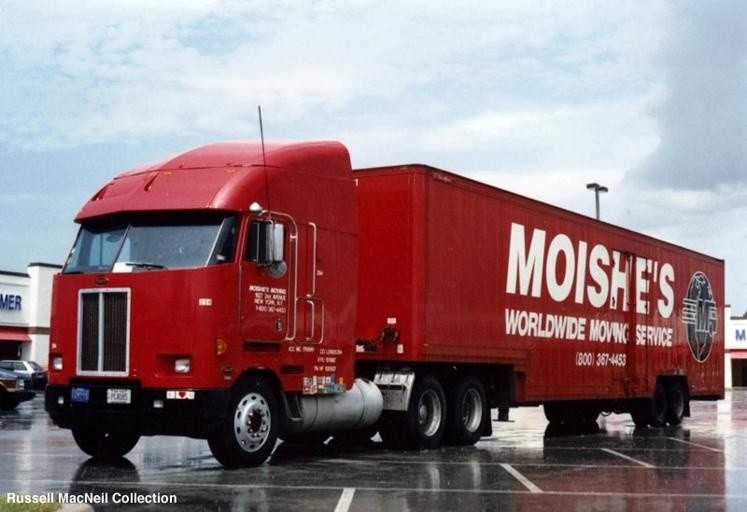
[{"xmin": 0, "ymin": 359, "xmax": 47, "ymax": 387}]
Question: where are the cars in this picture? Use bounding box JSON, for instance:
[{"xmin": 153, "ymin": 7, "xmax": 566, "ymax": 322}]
[{"xmin": 0, "ymin": 368, "xmax": 35, "ymax": 411}]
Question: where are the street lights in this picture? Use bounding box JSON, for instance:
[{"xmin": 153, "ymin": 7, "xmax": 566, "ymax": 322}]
[{"xmin": 584, "ymin": 181, "xmax": 609, "ymax": 219}]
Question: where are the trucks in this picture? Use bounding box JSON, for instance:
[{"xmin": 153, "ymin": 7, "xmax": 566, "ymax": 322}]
[{"xmin": 44, "ymin": 139, "xmax": 723, "ymax": 470}]
